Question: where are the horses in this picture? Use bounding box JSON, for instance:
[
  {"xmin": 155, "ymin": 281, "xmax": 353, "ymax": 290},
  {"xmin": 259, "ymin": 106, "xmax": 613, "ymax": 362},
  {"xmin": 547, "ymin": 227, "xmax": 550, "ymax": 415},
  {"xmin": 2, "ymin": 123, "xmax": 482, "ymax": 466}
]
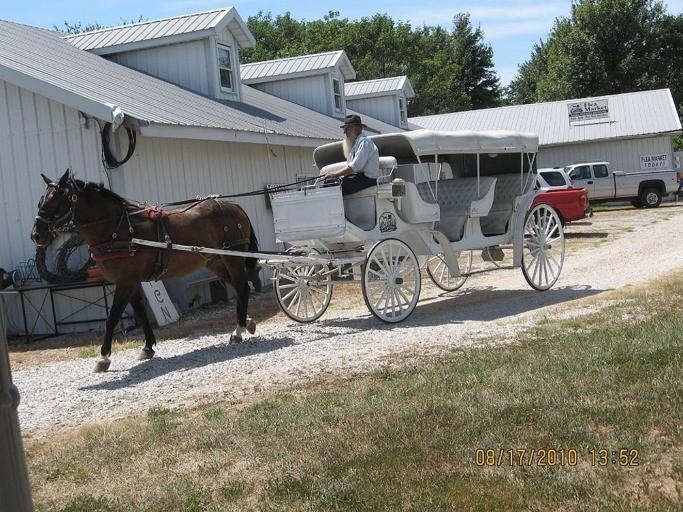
[{"xmin": 30, "ymin": 168, "xmax": 261, "ymax": 373}]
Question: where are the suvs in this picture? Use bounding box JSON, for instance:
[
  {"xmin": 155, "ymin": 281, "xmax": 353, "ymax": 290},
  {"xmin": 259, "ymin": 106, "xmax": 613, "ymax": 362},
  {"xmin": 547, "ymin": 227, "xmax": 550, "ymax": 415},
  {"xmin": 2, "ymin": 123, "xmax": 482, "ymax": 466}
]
[{"xmin": 534, "ymin": 167, "xmax": 575, "ymax": 194}]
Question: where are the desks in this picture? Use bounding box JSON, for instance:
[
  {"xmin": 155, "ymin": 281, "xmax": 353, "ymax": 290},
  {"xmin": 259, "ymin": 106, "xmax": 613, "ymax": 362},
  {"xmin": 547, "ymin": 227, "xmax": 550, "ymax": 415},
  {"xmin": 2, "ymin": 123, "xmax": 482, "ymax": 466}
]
[{"xmin": 17, "ymin": 278, "xmax": 139, "ymax": 344}]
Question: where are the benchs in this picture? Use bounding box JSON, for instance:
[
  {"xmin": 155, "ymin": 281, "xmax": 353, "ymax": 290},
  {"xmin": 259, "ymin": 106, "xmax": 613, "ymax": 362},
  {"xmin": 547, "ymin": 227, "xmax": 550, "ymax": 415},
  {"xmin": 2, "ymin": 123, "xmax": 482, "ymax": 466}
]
[{"xmin": 314, "ymin": 156, "xmax": 536, "ymax": 241}]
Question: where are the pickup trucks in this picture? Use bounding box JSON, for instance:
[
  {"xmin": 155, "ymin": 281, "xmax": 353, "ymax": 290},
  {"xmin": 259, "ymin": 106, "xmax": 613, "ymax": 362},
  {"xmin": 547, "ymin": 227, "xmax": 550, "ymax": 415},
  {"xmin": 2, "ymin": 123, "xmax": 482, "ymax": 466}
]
[
  {"xmin": 526, "ymin": 187, "xmax": 592, "ymax": 234},
  {"xmin": 562, "ymin": 161, "xmax": 679, "ymax": 210}
]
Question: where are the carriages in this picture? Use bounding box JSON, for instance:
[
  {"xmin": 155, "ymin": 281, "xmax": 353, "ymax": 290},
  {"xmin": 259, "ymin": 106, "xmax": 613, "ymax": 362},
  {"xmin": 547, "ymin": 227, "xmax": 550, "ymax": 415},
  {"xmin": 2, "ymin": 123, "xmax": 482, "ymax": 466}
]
[{"xmin": 27, "ymin": 127, "xmax": 566, "ymax": 375}]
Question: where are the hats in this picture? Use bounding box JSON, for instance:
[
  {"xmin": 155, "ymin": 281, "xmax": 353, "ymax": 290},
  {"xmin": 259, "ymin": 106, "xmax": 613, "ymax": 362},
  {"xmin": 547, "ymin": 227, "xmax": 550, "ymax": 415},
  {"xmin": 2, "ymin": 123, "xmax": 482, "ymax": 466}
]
[{"xmin": 340, "ymin": 114, "xmax": 368, "ymax": 129}]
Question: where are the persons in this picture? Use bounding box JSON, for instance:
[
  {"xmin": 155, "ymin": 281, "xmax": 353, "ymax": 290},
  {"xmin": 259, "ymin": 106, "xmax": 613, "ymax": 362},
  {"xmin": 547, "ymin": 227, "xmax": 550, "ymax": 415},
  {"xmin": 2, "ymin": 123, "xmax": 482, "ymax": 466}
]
[{"xmin": 317, "ymin": 114, "xmax": 379, "ymax": 196}]
